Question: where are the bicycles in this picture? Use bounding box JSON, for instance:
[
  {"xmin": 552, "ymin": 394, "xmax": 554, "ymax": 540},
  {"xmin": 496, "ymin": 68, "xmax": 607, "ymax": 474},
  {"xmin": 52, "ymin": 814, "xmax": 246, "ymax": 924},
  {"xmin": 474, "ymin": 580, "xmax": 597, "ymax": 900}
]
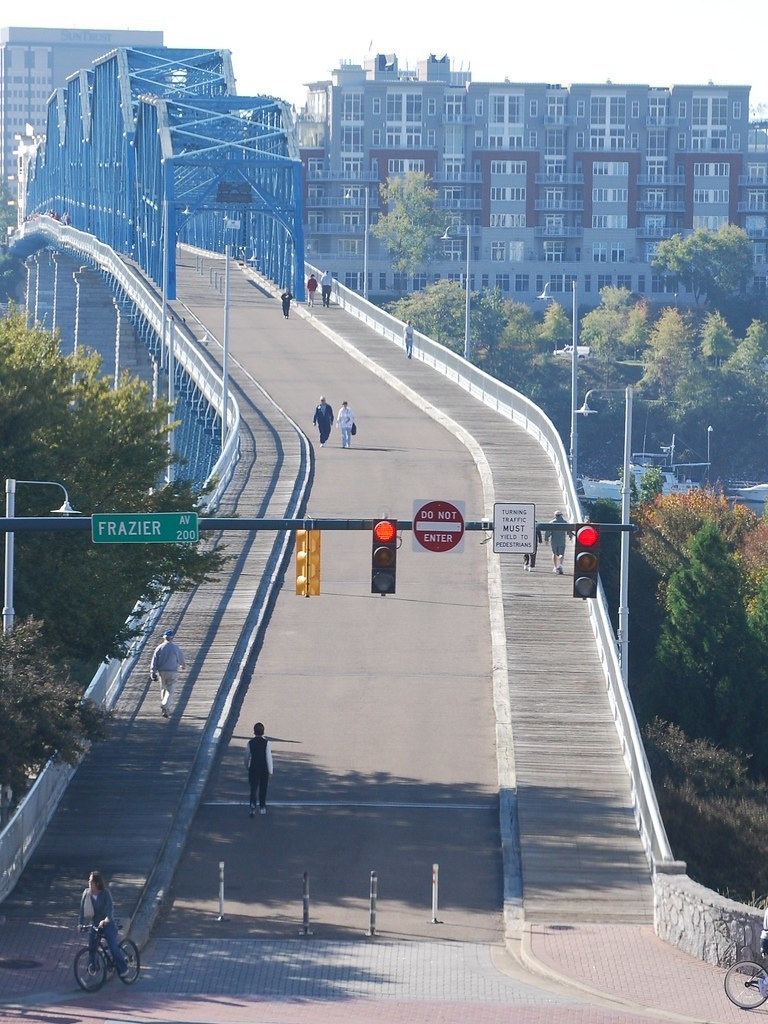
[
  {"xmin": 724, "ymin": 948, "xmax": 768, "ymax": 1010},
  {"xmin": 74, "ymin": 923, "xmax": 140, "ymax": 992}
]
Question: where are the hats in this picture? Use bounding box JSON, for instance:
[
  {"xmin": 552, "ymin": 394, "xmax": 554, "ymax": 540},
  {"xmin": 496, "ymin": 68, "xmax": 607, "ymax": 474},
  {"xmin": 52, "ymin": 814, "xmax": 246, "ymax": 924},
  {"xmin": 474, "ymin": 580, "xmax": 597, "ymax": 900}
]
[
  {"xmin": 320, "ymin": 396, "xmax": 325, "ymax": 401},
  {"xmin": 163, "ymin": 630, "xmax": 174, "ymax": 636},
  {"xmin": 555, "ymin": 510, "xmax": 563, "ymax": 515}
]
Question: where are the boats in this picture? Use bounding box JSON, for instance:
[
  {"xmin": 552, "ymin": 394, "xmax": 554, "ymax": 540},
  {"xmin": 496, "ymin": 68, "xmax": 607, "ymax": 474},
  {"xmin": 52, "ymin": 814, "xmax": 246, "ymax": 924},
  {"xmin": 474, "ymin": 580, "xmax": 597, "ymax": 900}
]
[
  {"xmin": 730, "ymin": 480, "xmax": 768, "ymax": 503},
  {"xmin": 579, "ymin": 413, "xmax": 712, "ymax": 502}
]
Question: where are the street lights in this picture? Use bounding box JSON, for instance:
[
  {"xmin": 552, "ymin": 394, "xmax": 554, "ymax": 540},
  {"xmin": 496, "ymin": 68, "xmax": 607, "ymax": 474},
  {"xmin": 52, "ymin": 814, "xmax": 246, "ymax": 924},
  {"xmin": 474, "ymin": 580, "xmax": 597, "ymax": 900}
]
[
  {"xmin": 573, "ymin": 383, "xmax": 632, "ymax": 685},
  {"xmin": 439, "ymin": 224, "xmax": 470, "ymax": 361},
  {"xmin": 2, "ymin": 477, "xmax": 82, "ymax": 679},
  {"xmin": 345, "ymin": 186, "xmax": 369, "ymax": 299},
  {"xmin": 166, "ymin": 316, "xmax": 215, "ymax": 484},
  {"xmin": 536, "ymin": 280, "xmax": 578, "ymax": 488},
  {"xmin": 161, "ymin": 200, "xmax": 194, "ymax": 370},
  {"xmin": 221, "ymin": 244, "xmax": 261, "ymax": 446}
]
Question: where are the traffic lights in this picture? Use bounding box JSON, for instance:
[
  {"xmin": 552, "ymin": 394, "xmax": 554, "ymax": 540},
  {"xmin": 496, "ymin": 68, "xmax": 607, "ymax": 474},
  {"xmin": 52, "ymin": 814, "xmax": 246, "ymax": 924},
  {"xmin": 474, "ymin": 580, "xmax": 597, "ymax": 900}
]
[
  {"xmin": 371, "ymin": 519, "xmax": 397, "ymax": 594},
  {"xmin": 573, "ymin": 523, "xmax": 600, "ymax": 598}
]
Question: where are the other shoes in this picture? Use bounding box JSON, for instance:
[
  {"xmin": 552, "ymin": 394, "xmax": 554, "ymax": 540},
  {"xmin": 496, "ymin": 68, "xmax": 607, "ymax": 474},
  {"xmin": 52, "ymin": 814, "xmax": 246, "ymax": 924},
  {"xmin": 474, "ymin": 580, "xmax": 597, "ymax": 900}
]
[
  {"xmin": 249, "ymin": 803, "xmax": 255, "ymax": 816},
  {"xmin": 161, "ymin": 706, "xmax": 169, "ymax": 717},
  {"xmin": 320, "ymin": 443, "xmax": 326, "ymax": 448},
  {"xmin": 553, "ymin": 565, "xmax": 563, "ymax": 574},
  {"xmin": 523, "ymin": 565, "xmax": 534, "ymax": 572},
  {"xmin": 120, "ymin": 968, "xmax": 130, "ymax": 977},
  {"xmin": 260, "ymin": 808, "xmax": 266, "ymax": 815},
  {"xmin": 342, "ymin": 444, "xmax": 350, "ymax": 448}
]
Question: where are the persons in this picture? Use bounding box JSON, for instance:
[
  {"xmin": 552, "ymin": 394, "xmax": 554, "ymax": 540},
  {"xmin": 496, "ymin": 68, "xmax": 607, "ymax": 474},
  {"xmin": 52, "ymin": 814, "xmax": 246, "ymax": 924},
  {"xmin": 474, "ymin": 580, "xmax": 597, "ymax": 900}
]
[
  {"xmin": 320, "ymin": 271, "xmax": 332, "ymax": 308},
  {"xmin": 77, "ymin": 872, "xmax": 134, "ymax": 982},
  {"xmin": 281, "ymin": 288, "xmax": 293, "ymax": 319},
  {"xmin": 336, "ymin": 401, "xmax": 354, "ymax": 449},
  {"xmin": 307, "ymin": 274, "xmax": 317, "ymax": 308},
  {"xmin": 545, "ymin": 511, "xmax": 572, "ymax": 575},
  {"xmin": 523, "ymin": 520, "xmax": 542, "ymax": 571},
  {"xmin": 150, "ymin": 630, "xmax": 185, "ymax": 718},
  {"xmin": 404, "ymin": 321, "xmax": 413, "ymax": 360},
  {"xmin": 244, "ymin": 723, "xmax": 273, "ymax": 817},
  {"xmin": 26, "ymin": 209, "xmax": 70, "ymax": 226},
  {"xmin": 313, "ymin": 396, "xmax": 333, "ymax": 447},
  {"xmin": 758, "ymin": 908, "xmax": 768, "ymax": 998}
]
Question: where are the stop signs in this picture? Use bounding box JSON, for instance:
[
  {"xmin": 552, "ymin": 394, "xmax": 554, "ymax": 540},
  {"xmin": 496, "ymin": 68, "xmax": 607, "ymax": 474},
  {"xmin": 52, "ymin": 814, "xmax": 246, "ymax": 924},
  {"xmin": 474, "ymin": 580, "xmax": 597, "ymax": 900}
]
[{"xmin": 411, "ymin": 498, "xmax": 465, "ymax": 554}]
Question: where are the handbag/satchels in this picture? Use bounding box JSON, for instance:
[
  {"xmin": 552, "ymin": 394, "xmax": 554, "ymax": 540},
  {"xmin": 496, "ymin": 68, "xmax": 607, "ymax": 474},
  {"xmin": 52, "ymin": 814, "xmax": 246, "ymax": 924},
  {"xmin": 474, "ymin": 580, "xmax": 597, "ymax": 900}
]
[{"xmin": 351, "ymin": 423, "xmax": 357, "ymax": 436}]
